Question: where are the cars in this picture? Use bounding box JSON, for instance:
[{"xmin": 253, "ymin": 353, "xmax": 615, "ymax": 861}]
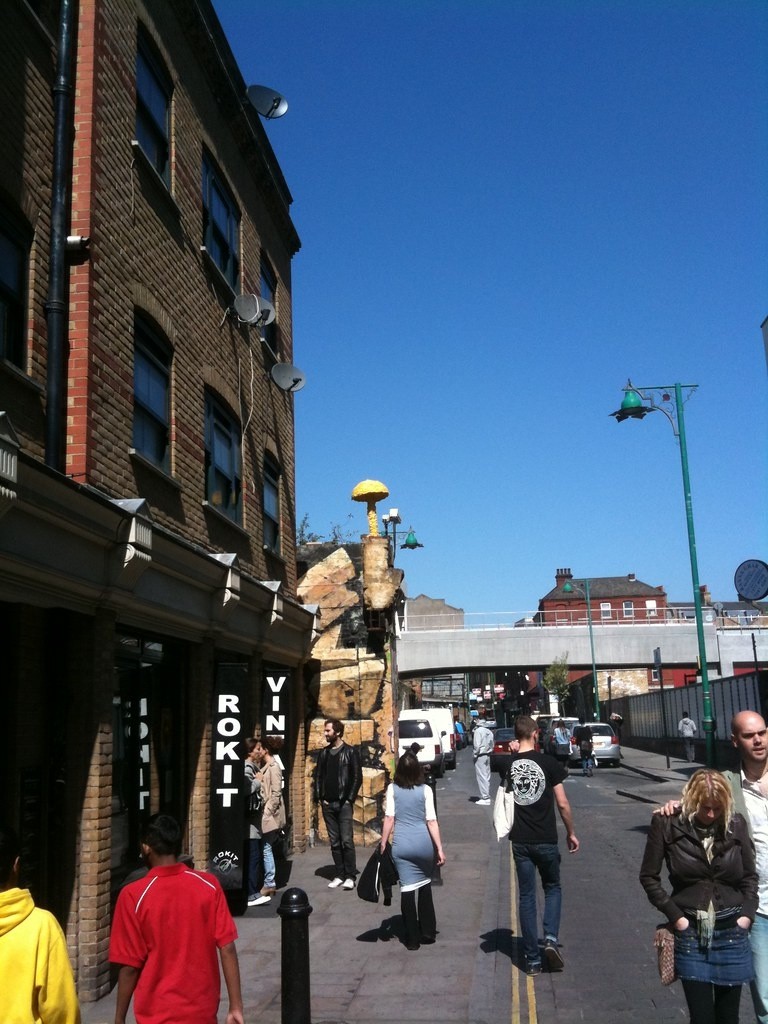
[
  {"xmin": 542, "ymin": 717, "xmax": 581, "ymax": 758},
  {"xmin": 568, "ymin": 722, "xmax": 621, "ymax": 768},
  {"xmin": 535, "ymin": 715, "xmax": 562, "ymax": 744},
  {"xmin": 487, "ymin": 728, "xmax": 540, "ymax": 771},
  {"xmin": 397, "ymin": 719, "xmax": 447, "ymax": 778},
  {"xmin": 455, "ymin": 721, "xmax": 468, "ymax": 749}
]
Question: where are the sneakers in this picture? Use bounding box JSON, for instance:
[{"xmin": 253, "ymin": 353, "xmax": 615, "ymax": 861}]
[
  {"xmin": 328, "ymin": 878, "xmax": 342, "ymax": 889},
  {"xmin": 343, "ymin": 879, "xmax": 354, "ymax": 889},
  {"xmin": 247, "ymin": 895, "xmax": 272, "ymax": 907},
  {"xmin": 475, "ymin": 799, "xmax": 490, "ymax": 805}
]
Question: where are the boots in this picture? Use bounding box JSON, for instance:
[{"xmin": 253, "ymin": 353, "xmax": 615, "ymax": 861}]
[
  {"xmin": 588, "ymin": 765, "xmax": 593, "ymax": 775},
  {"xmin": 583, "ymin": 769, "xmax": 587, "ymax": 776}
]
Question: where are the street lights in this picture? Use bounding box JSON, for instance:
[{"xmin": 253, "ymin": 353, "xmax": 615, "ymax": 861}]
[
  {"xmin": 561, "ymin": 577, "xmax": 602, "ymax": 723},
  {"xmin": 608, "ymin": 379, "xmax": 719, "ymax": 771}
]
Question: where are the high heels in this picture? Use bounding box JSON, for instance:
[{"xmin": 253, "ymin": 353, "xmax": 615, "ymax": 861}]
[{"xmin": 260, "ymin": 886, "xmax": 276, "ymax": 896}]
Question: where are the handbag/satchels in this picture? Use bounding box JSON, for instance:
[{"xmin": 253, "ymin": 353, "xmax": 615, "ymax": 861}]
[
  {"xmin": 492, "ymin": 753, "xmax": 516, "ymax": 842},
  {"xmin": 654, "ymin": 922, "xmax": 679, "ymax": 985},
  {"xmin": 580, "ymin": 741, "xmax": 590, "ymax": 751}
]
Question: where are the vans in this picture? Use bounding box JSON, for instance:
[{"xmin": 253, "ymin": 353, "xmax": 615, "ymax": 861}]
[{"xmin": 397, "ymin": 708, "xmax": 456, "ymax": 770}]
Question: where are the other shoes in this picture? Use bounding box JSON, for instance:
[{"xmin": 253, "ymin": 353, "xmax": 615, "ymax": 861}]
[
  {"xmin": 424, "ymin": 935, "xmax": 435, "ymax": 942},
  {"xmin": 544, "ymin": 941, "xmax": 564, "ymax": 971},
  {"xmin": 527, "ymin": 963, "xmax": 542, "ymax": 976},
  {"xmin": 408, "ymin": 945, "xmax": 419, "ymax": 950}
]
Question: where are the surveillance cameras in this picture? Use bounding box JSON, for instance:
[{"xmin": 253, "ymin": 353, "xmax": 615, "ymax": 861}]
[{"xmin": 390, "ymin": 509, "xmax": 402, "ymax": 524}]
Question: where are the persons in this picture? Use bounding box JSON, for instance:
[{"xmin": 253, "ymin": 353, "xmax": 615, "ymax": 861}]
[
  {"xmin": 652, "ymin": 710, "xmax": 768, "ymax": 1024},
  {"xmin": 110, "ymin": 813, "xmax": 244, "ymax": 1024},
  {"xmin": 472, "ymin": 715, "xmax": 494, "ymax": 805},
  {"xmin": 575, "ymin": 716, "xmax": 593, "ymax": 777},
  {"xmin": 258, "ymin": 736, "xmax": 286, "ymax": 897},
  {"xmin": 0, "ymin": 834, "xmax": 81, "ymax": 1024},
  {"xmin": 640, "ymin": 770, "xmax": 759, "ymax": 1024},
  {"xmin": 240, "ymin": 738, "xmax": 271, "ymax": 906},
  {"xmin": 607, "ymin": 713, "xmax": 624, "ymax": 759},
  {"xmin": 380, "ymin": 753, "xmax": 446, "ymax": 949},
  {"xmin": 553, "ymin": 719, "xmax": 573, "ymax": 772},
  {"xmin": 471, "ymin": 717, "xmax": 476, "ymax": 741},
  {"xmin": 500, "ymin": 716, "xmax": 580, "ymax": 976},
  {"xmin": 317, "ymin": 719, "xmax": 363, "ymax": 889},
  {"xmin": 678, "ymin": 711, "xmax": 697, "ymax": 763}
]
